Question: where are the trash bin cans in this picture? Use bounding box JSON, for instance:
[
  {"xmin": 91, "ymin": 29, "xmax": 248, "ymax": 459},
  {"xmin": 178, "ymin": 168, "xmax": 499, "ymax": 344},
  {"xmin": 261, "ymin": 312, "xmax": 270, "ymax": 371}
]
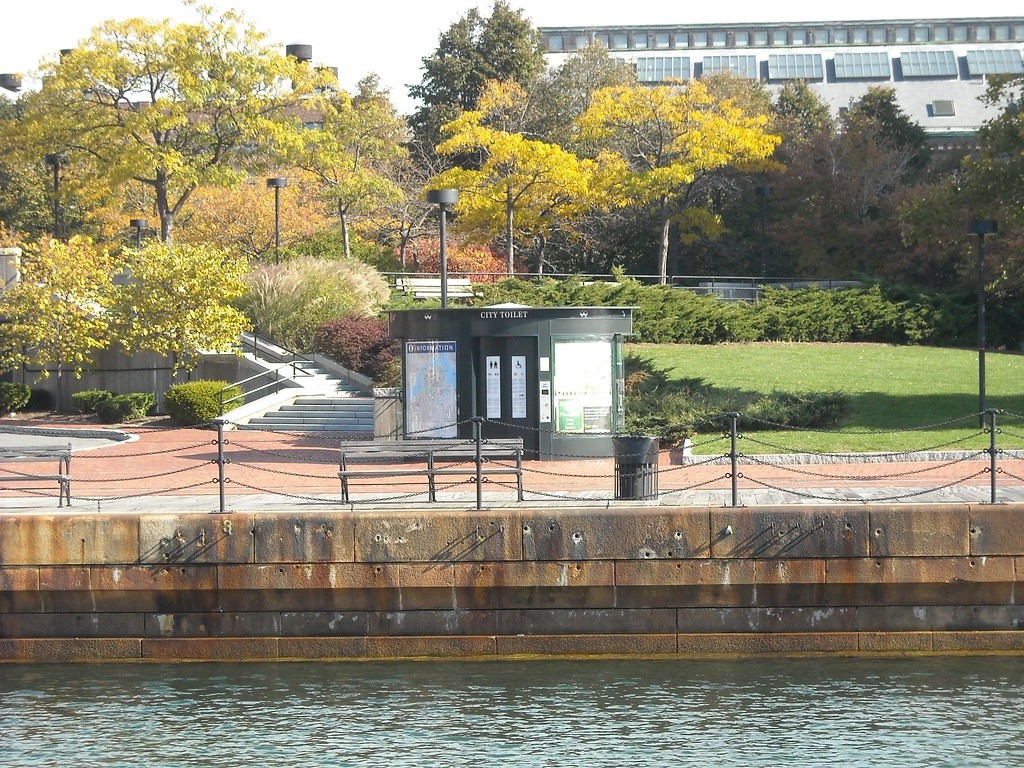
[{"xmin": 610, "ymin": 433, "xmax": 663, "ymax": 502}]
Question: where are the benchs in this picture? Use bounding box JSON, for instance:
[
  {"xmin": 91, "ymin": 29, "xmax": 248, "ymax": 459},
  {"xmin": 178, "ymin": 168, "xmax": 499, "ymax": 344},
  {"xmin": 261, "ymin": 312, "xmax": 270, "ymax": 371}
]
[{"xmin": 337, "ymin": 439, "xmax": 525, "ymax": 504}]
